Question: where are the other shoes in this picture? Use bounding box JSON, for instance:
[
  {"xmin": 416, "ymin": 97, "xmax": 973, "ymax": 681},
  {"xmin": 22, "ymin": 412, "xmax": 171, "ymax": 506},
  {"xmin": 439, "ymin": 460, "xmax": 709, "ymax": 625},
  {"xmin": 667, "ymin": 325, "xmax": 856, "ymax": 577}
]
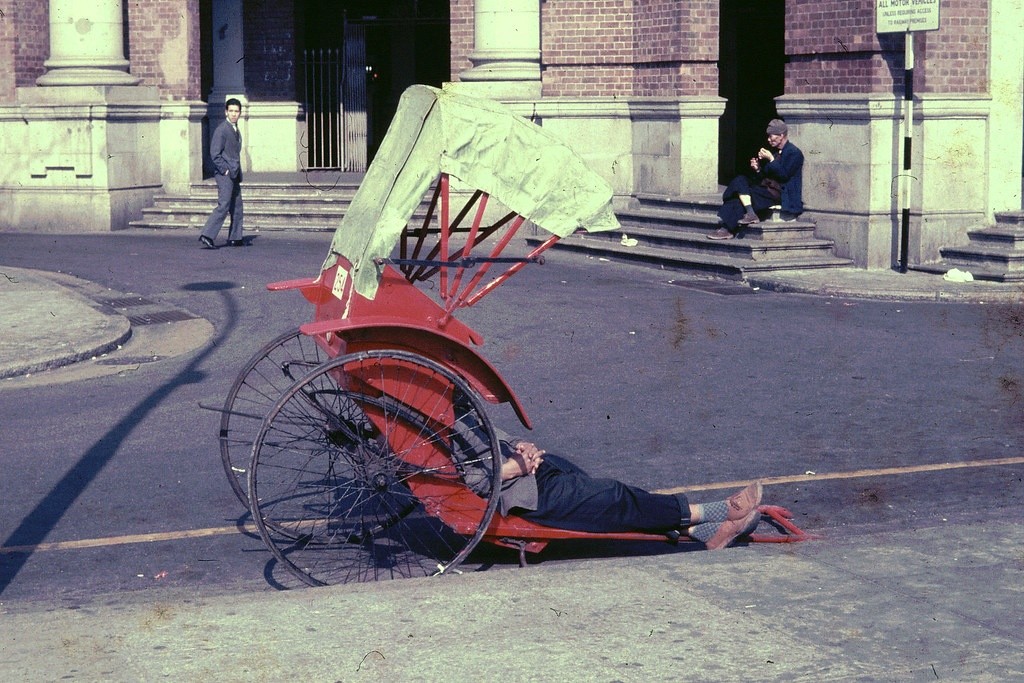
[
  {"xmin": 706, "ymin": 226, "xmax": 734, "ymax": 240},
  {"xmin": 705, "ymin": 510, "xmax": 761, "ymax": 550},
  {"xmin": 725, "ymin": 481, "xmax": 763, "ymax": 520},
  {"xmin": 737, "ymin": 213, "xmax": 761, "ymax": 225}
]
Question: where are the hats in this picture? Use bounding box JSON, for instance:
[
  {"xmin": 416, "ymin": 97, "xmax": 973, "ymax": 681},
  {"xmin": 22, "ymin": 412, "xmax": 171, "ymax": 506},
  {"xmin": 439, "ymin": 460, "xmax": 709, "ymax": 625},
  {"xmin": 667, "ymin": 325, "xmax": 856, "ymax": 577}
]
[{"xmin": 766, "ymin": 119, "xmax": 788, "ymax": 134}]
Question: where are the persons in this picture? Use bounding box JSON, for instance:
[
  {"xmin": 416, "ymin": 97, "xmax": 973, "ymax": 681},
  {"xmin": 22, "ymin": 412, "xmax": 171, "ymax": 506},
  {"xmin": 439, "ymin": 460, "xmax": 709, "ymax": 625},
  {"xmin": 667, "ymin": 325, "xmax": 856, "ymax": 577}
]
[
  {"xmin": 449, "ymin": 383, "xmax": 764, "ymax": 553},
  {"xmin": 706, "ymin": 118, "xmax": 804, "ymax": 238},
  {"xmin": 199, "ymin": 98, "xmax": 253, "ymax": 248}
]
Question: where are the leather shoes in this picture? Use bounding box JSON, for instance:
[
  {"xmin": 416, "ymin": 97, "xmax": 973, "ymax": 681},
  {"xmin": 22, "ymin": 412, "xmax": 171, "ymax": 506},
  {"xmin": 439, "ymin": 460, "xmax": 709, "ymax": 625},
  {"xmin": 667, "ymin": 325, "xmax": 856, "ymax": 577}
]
[
  {"xmin": 230, "ymin": 239, "xmax": 252, "ymax": 246},
  {"xmin": 199, "ymin": 235, "xmax": 220, "ymax": 249}
]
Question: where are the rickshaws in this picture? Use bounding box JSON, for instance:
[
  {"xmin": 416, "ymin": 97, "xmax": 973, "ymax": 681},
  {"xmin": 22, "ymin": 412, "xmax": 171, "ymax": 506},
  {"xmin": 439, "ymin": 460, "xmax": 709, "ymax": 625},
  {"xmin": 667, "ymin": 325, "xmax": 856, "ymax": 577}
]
[{"xmin": 213, "ymin": 82, "xmax": 818, "ymax": 586}]
